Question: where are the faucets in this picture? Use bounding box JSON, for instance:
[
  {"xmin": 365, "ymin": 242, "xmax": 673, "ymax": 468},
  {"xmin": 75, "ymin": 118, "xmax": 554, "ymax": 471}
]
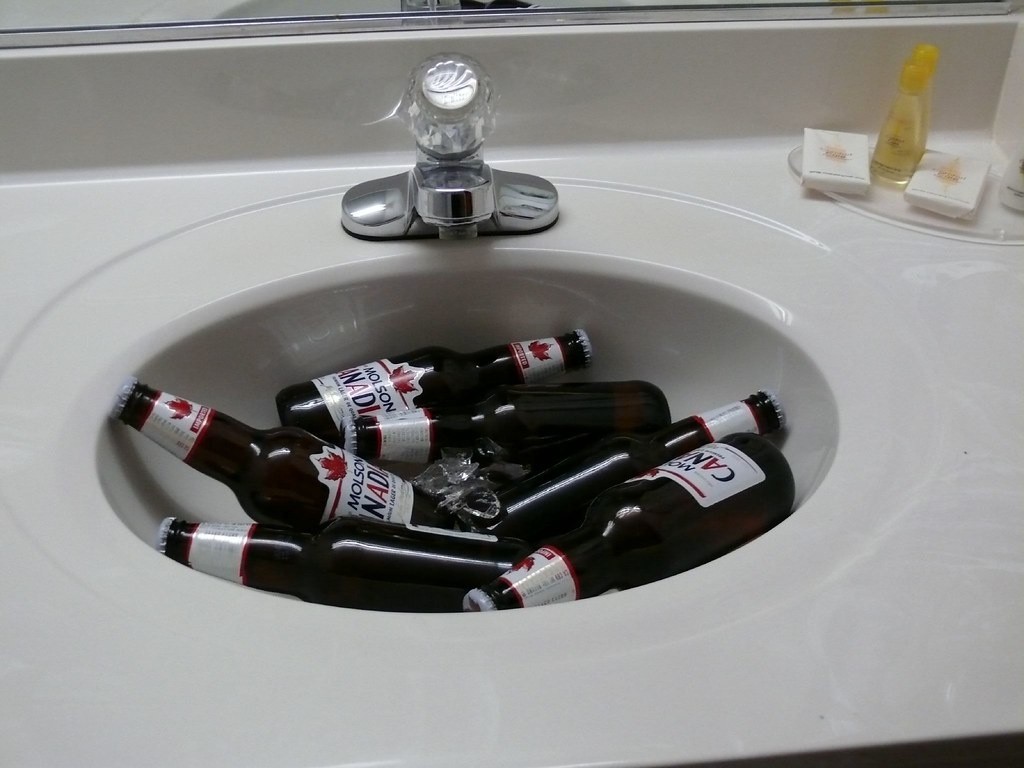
[{"xmin": 398, "ymin": 46, "xmax": 506, "ymax": 249}]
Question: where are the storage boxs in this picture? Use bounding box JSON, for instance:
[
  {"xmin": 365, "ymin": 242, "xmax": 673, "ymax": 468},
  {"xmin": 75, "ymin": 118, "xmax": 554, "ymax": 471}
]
[
  {"xmin": 904, "ymin": 149, "xmax": 990, "ymax": 220},
  {"xmin": 799, "ymin": 128, "xmax": 872, "ymax": 196}
]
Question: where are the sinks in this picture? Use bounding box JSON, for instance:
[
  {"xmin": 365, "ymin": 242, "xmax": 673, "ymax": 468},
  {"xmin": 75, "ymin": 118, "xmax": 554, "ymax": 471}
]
[{"xmin": 66, "ymin": 234, "xmax": 863, "ymax": 687}]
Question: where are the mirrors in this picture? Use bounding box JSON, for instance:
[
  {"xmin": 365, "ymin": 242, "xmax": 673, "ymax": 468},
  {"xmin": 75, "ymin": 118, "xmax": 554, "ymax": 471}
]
[{"xmin": 0, "ymin": 0, "xmax": 1010, "ymax": 46}]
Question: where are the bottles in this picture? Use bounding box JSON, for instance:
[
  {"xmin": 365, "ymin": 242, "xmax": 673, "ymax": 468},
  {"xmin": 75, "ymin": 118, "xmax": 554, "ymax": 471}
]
[
  {"xmin": 463, "ymin": 433, "xmax": 796, "ymax": 611},
  {"xmin": 275, "ymin": 329, "xmax": 593, "ymax": 447},
  {"xmin": 455, "ymin": 389, "xmax": 786, "ymax": 543},
  {"xmin": 340, "ymin": 379, "xmax": 671, "ymax": 466},
  {"xmin": 157, "ymin": 516, "xmax": 529, "ymax": 612},
  {"xmin": 110, "ymin": 377, "xmax": 452, "ymax": 532}
]
